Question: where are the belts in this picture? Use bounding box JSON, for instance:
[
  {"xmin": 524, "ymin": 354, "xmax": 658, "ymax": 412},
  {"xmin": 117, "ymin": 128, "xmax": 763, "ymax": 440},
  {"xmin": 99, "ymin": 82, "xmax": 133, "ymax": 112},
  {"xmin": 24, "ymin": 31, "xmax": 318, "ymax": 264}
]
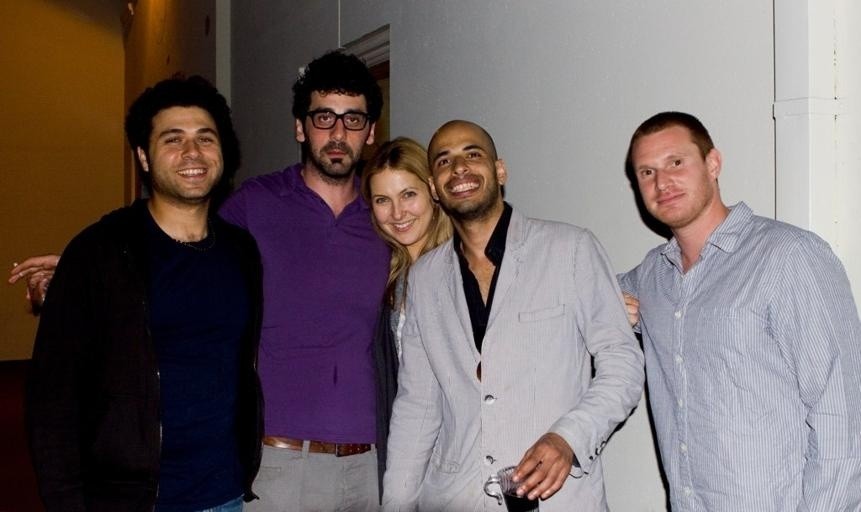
[{"xmin": 260, "ymin": 433, "xmax": 374, "ymax": 458}]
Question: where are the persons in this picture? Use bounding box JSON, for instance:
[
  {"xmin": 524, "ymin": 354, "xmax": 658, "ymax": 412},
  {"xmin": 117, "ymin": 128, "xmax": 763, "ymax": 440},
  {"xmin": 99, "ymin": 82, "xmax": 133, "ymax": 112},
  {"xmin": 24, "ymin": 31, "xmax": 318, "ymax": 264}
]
[
  {"xmin": 613, "ymin": 111, "xmax": 860, "ymax": 512},
  {"xmin": 20, "ymin": 67, "xmax": 267, "ymax": 511},
  {"xmin": 7, "ymin": 45, "xmax": 398, "ymax": 511}
]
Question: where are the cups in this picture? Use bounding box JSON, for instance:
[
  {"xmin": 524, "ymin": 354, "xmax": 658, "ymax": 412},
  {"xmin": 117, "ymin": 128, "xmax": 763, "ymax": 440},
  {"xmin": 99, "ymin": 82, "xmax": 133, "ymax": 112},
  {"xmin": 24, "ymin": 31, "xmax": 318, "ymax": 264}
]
[{"xmin": 482, "ymin": 466, "xmax": 540, "ymax": 512}]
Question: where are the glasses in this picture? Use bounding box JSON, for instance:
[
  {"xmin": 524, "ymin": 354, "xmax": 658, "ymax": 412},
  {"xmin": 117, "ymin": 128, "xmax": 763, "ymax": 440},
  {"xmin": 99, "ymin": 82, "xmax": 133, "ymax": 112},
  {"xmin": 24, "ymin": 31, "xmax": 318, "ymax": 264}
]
[{"xmin": 301, "ymin": 107, "xmax": 371, "ymax": 132}]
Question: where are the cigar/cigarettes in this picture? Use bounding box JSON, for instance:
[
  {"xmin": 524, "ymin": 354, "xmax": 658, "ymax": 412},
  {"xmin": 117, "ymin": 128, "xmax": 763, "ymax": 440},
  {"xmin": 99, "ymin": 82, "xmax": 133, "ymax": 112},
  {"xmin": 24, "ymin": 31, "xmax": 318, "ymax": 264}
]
[{"xmin": 13, "ymin": 262, "xmax": 23, "ymax": 276}]
